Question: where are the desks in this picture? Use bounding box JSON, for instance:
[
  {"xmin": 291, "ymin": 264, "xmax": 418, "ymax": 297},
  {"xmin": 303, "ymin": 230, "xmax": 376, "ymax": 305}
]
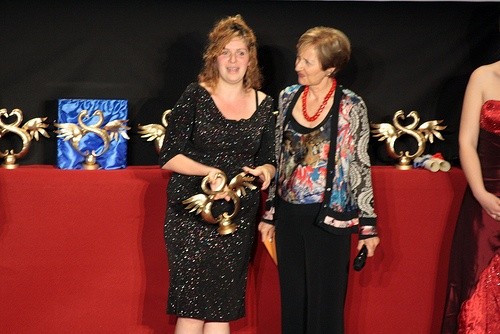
[{"xmin": 0, "ymin": 166, "xmax": 469, "ymax": 334}]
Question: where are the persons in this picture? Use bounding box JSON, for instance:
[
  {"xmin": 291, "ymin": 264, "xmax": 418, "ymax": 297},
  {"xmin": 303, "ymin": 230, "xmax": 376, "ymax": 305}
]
[
  {"xmin": 159, "ymin": 14, "xmax": 278, "ymax": 334},
  {"xmin": 441, "ymin": 60, "xmax": 500, "ymax": 334},
  {"xmin": 258, "ymin": 26, "xmax": 381, "ymax": 334}
]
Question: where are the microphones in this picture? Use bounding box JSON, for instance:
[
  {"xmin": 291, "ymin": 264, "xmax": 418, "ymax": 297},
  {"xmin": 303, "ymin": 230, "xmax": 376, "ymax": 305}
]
[{"xmin": 353, "ymin": 246, "xmax": 368, "ymax": 271}]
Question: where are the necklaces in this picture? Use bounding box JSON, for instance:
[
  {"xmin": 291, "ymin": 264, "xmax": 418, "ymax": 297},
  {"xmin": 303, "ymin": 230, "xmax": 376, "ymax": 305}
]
[{"xmin": 300, "ymin": 77, "xmax": 336, "ymax": 122}]
